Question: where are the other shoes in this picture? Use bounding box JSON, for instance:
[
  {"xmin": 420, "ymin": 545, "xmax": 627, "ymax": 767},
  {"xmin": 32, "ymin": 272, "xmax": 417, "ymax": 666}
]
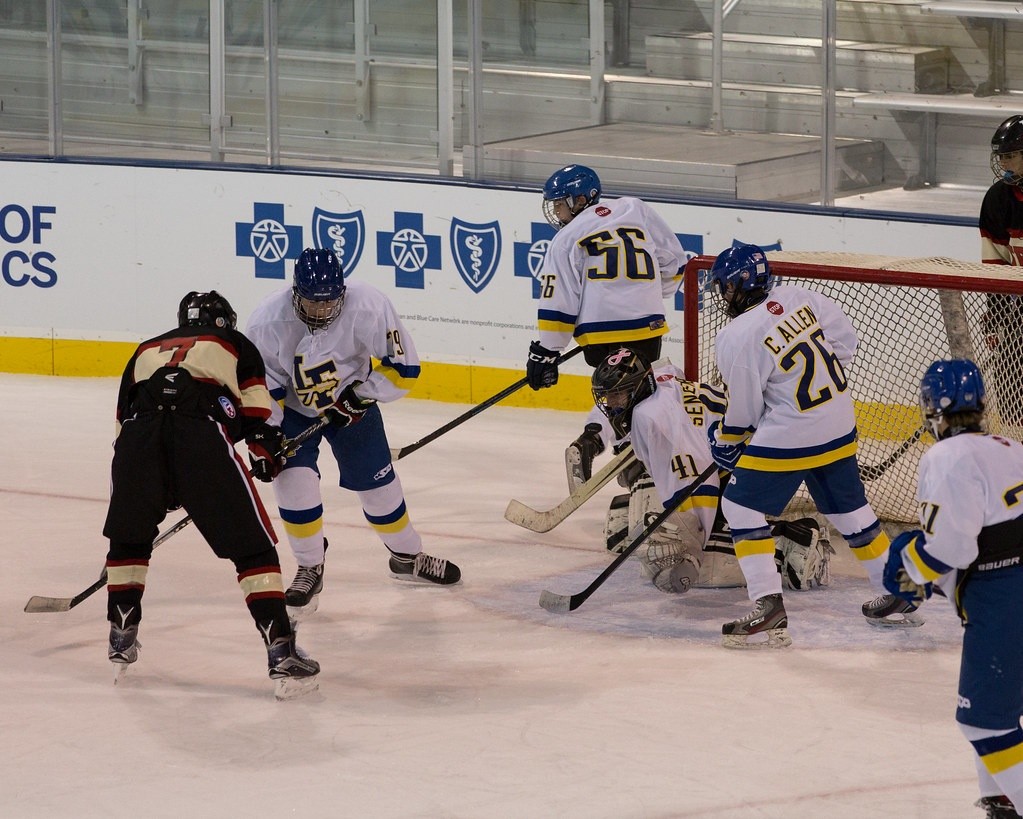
[
  {"xmin": 783, "ymin": 518, "xmax": 835, "ymax": 591},
  {"xmin": 973, "ymin": 796, "xmax": 1022, "ymax": 819}
]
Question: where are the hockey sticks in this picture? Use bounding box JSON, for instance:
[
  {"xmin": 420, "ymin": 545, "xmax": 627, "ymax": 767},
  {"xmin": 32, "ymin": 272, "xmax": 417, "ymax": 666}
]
[
  {"xmin": 384, "ymin": 345, "xmax": 583, "ymax": 461},
  {"xmin": 504, "ymin": 442, "xmax": 636, "ymax": 533},
  {"xmin": 536, "ymin": 460, "xmax": 719, "ymax": 618},
  {"xmin": 24, "ymin": 418, "xmax": 325, "ymax": 613}
]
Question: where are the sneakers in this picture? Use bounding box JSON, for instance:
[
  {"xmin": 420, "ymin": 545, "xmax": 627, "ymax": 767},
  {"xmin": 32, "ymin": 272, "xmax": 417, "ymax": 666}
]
[
  {"xmin": 109, "ymin": 603, "xmax": 142, "ymax": 686},
  {"xmin": 722, "ymin": 593, "xmax": 792, "ymax": 649},
  {"xmin": 257, "ymin": 619, "xmax": 320, "ymax": 700},
  {"xmin": 862, "ymin": 595, "xmax": 925, "ymax": 629},
  {"xmin": 565, "ymin": 423, "xmax": 605, "ymax": 494},
  {"xmin": 285, "ymin": 537, "xmax": 329, "ymax": 617},
  {"xmin": 385, "ymin": 543, "xmax": 462, "ymax": 586}
]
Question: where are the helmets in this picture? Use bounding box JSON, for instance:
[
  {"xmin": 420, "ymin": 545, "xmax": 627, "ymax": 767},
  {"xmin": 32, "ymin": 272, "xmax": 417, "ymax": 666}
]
[
  {"xmin": 178, "ymin": 291, "xmax": 237, "ymax": 329},
  {"xmin": 710, "ymin": 246, "xmax": 769, "ymax": 316},
  {"xmin": 542, "ymin": 165, "xmax": 601, "ymax": 231},
  {"xmin": 293, "ymin": 248, "xmax": 345, "ymax": 329},
  {"xmin": 591, "ymin": 349, "xmax": 656, "ymax": 438},
  {"xmin": 990, "ymin": 115, "xmax": 1023, "ymax": 185},
  {"xmin": 921, "ymin": 359, "xmax": 985, "ymax": 417}
]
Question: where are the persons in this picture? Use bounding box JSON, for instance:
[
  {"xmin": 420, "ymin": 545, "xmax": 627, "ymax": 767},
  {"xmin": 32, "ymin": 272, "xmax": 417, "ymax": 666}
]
[
  {"xmin": 883, "ymin": 361, "xmax": 1023, "ymax": 819},
  {"xmin": 241, "ymin": 248, "xmax": 463, "ymax": 618},
  {"xmin": 979, "ymin": 115, "xmax": 1023, "ymax": 426},
  {"xmin": 589, "ymin": 347, "xmax": 836, "ymax": 592},
  {"xmin": 710, "ymin": 244, "xmax": 926, "ymax": 647},
  {"xmin": 526, "ymin": 164, "xmax": 687, "ymax": 496},
  {"xmin": 103, "ymin": 291, "xmax": 322, "ymax": 700}
]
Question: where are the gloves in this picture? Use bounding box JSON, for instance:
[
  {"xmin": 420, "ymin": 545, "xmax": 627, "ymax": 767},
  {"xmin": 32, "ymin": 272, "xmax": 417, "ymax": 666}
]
[
  {"xmin": 883, "ymin": 530, "xmax": 934, "ymax": 609},
  {"xmin": 245, "ymin": 423, "xmax": 288, "ymax": 482},
  {"xmin": 707, "ymin": 420, "xmax": 746, "ymax": 472},
  {"xmin": 325, "ymin": 381, "xmax": 377, "ymax": 429},
  {"xmin": 527, "ymin": 341, "xmax": 559, "ymax": 390}
]
